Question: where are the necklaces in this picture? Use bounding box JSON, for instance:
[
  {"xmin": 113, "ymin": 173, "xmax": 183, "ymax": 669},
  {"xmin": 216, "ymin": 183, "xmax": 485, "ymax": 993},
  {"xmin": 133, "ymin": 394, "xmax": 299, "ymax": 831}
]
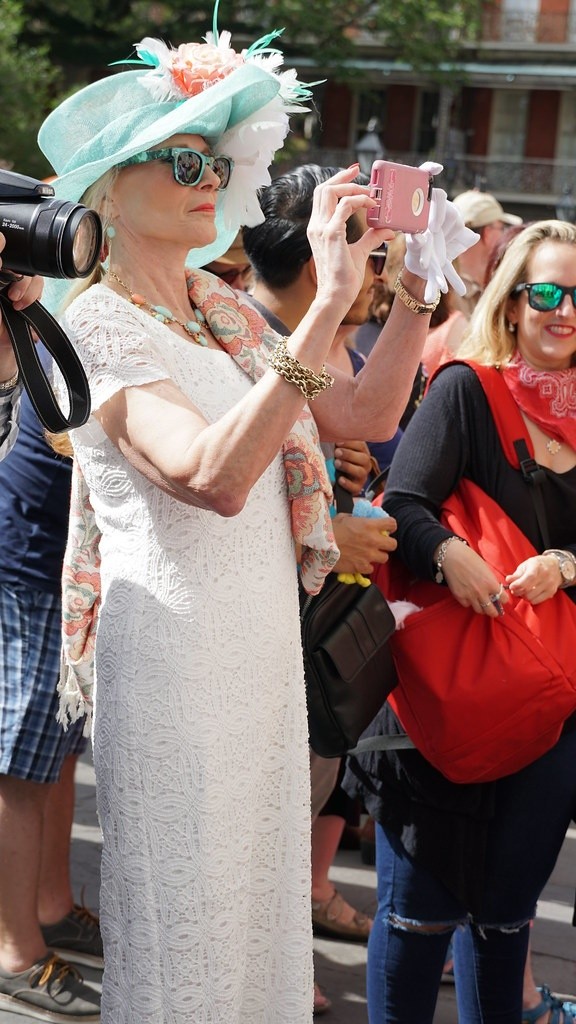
[
  {"xmin": 105, "ymin": 267, "xmax": 211, "ymax": 346},
  {"xmin": 547, "ymin": 439, "xmax": 560, "ymax": 454}
]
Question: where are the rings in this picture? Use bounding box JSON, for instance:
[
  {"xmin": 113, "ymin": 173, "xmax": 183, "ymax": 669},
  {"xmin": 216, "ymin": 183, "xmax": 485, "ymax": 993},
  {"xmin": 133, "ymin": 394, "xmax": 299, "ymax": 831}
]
[{"xmin": 483, "ymin": 603, "xmax": 489, "ymax": 607}]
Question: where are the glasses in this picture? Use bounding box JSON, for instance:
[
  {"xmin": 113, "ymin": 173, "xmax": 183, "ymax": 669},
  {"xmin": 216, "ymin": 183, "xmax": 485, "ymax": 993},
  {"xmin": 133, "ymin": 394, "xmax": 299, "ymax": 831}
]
[
  {"xmin": 111, "ymin": 147, "xmax": 235, "ymax": 192},
  {"xmin": 509, "ymin": 282, "xmax": 576, "ymax": 312},
  {"xmin": 368, "ymin": 241, "xmax": 389, "ymax": 274}
]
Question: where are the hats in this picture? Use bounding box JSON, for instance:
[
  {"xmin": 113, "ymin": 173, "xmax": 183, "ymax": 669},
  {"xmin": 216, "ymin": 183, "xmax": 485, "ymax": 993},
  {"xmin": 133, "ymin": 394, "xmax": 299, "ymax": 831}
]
[
  {"xmin": 452, "ymin": 189, "xmax": 524, "ymax": 229},
  {"xmin": 37, "ymin": 0, "xmax": 327, "ymax": 317}
]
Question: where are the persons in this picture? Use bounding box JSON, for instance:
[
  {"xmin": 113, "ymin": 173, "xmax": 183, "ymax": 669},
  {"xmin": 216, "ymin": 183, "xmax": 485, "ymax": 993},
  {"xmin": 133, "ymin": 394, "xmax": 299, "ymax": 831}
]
[
  {"xmin": 0, "ymin": 210, "xmax": 249, "ymax": 1015},
  {"xmin": 368, "ymin": 220, "xmax": 576, "ymax": 1023},
  {"xmin": 240, "ymin": 165, "xmax": 576, "ymax": 1012},
  {"xmin": 38, "ymin": 31, "xmax": 444, "ymax": 1024}
]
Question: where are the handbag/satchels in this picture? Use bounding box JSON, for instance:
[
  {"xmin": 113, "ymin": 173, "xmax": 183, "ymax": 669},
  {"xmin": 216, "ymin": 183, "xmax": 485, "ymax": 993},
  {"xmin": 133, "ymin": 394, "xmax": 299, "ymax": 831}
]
[{"xmin": 294, "ymin": 560, "xmax": 395, "ymax": 759}]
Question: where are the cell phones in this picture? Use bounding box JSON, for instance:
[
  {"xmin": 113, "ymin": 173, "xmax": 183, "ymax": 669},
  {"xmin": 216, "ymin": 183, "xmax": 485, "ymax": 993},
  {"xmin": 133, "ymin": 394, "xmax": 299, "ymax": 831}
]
[{"xmin": 365, "ymin": 160, "xmax": 434, "ymax": 234}]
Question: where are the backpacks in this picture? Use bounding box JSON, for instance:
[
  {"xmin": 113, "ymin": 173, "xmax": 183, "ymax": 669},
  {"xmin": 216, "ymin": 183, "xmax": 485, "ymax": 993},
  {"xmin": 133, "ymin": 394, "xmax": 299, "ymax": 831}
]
[{"xmin": 360, "ymin": 356, "xmax": 575, "ymax": 782}]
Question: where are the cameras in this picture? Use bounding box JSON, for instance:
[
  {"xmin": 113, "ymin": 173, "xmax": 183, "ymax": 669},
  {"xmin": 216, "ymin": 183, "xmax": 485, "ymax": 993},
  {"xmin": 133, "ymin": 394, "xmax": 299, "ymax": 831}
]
[{"xmin": 0, "ymin": 170, "xmax": 102, "ymax": 280}]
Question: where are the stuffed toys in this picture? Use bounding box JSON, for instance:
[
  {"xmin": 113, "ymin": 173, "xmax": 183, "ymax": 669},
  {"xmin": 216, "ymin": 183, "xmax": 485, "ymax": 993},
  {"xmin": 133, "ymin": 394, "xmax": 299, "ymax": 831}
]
[{"xmin": 336, "ymin": 500, "xmax": 390, "ymax": 588}]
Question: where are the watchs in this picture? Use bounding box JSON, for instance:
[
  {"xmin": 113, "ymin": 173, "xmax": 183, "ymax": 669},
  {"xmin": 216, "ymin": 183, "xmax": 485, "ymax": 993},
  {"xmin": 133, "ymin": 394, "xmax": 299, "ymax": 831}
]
[{"xmin": 550, "ymin": 551, "xmax": 576, "ymax": 588}]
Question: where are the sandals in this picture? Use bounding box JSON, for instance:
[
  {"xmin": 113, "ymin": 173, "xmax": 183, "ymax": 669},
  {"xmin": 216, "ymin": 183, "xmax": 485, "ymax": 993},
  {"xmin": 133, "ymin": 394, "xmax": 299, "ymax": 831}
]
[
  {"xmin": 440, "ymin": 948, "xmax": 456, "ymax": 982},
  {"xmin": 312, "ymin": 991, "xmax": 332, "ymax": 1011},
  {"xmin": 310, "ymin": 885, "xmax": 374, "ymax": 945},
  {"xmin": 521, "ymin": 983, "xmax": 576, "ymax": 1024}
]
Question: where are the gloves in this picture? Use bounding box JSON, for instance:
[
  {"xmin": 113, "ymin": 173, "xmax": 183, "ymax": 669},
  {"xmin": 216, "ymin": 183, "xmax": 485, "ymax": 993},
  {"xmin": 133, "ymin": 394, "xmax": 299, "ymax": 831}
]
[{"xmin": 404, "ymin": 161, "xmax": 481, "ymax": 304}]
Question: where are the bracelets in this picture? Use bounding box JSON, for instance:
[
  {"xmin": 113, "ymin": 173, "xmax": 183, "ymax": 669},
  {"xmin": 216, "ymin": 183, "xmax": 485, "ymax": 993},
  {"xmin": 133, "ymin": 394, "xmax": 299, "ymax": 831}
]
[
  {"xmin": 436, "ymin": 537, "xmax": 466, "ymax": 583},
  {"xmin": 265, "ymin": 340, "xmax": 334, "ymax": 398},
  {"xmin": 395, "ymin": 276, "xmax": 441, "ymax": 313},
  {"xmin": 492, "ymin": 585, "xmax": 502, "ymax": 602},
  {"xmin": 543, "ymin": 549, "xmax": 576, "ymax": 564}
]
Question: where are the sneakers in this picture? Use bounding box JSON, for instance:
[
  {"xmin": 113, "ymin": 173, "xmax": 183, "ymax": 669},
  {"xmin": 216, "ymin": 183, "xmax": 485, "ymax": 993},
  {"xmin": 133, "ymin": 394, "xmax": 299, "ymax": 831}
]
[
  {"xmin": 0, "ymin": 951, "xmax": 104, "ymax": 1023},
  {"xmin": 41, "ymin": 885, "xmax": 104, "ymax": 958}
]
